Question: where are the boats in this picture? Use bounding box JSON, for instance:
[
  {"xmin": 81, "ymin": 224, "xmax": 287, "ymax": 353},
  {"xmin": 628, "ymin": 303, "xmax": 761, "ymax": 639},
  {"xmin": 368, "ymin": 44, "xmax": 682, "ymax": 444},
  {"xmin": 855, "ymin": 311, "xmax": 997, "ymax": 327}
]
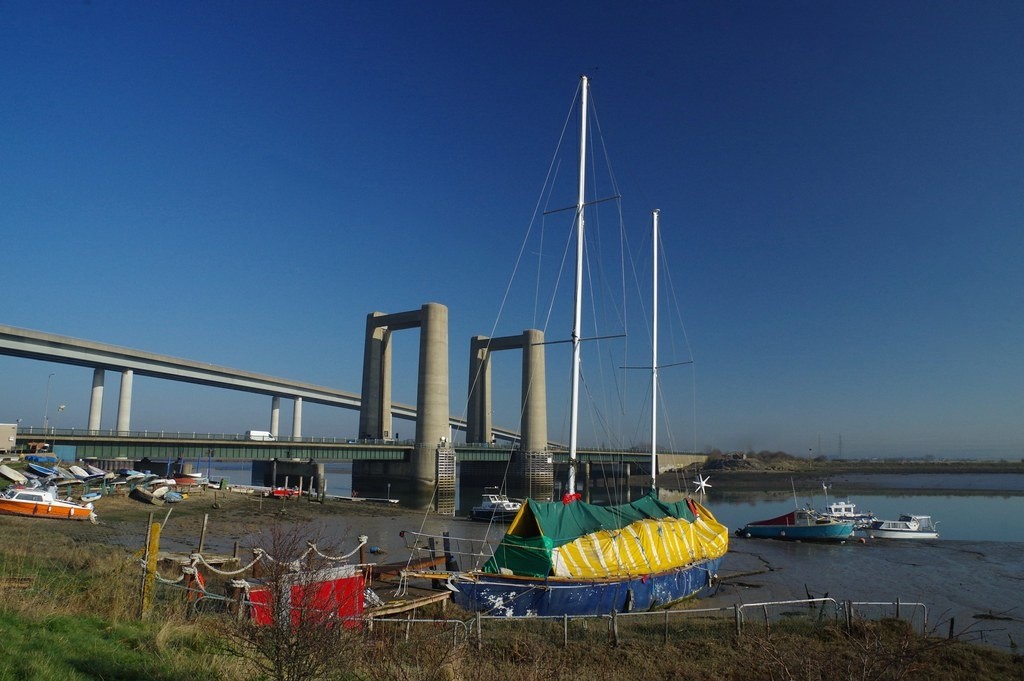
[
  {"xmin": 466, "ymin": 493, "xmax": 522, "ymax": 523},
  {"xmin": 0, "ymin": 481, "xmax": 93, "ymax": 521},
  {"xmin": 862, "ymin": 513, "xmax": 940, "ymax": 540},
  {"xmin": 735, "ymin": 475, "xmax": 855, "ymax": 541},
  {"xmin": 0, "ymin": 449, "xmax": 222, "ymax": 503},
  {"xmin": 820, "ymin": 481, "xmax": 880, "ymax": 538}
]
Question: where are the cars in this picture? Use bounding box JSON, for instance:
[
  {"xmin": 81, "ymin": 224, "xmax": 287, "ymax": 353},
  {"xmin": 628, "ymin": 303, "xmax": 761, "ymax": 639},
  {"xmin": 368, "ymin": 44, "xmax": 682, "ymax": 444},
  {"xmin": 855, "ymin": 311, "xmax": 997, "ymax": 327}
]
[{"xmin": 346, "ymin": 439, "xmax": 361, "ymax": 444}]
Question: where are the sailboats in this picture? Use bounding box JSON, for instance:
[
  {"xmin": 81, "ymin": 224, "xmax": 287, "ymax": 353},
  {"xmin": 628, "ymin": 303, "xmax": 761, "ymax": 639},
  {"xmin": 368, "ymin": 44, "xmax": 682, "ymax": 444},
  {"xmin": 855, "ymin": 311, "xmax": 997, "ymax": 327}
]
[{"xmin": 402, "ymin": 73, "xmax": 731, "ymax": 620}]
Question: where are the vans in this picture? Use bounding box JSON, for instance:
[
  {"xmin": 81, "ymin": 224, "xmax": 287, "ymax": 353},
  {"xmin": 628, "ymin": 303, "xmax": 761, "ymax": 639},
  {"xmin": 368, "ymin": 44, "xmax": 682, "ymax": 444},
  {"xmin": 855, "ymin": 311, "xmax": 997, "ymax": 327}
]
[{"xmin": 245, "ymin": 430, "xmax": 275, "ymax": 442}]
